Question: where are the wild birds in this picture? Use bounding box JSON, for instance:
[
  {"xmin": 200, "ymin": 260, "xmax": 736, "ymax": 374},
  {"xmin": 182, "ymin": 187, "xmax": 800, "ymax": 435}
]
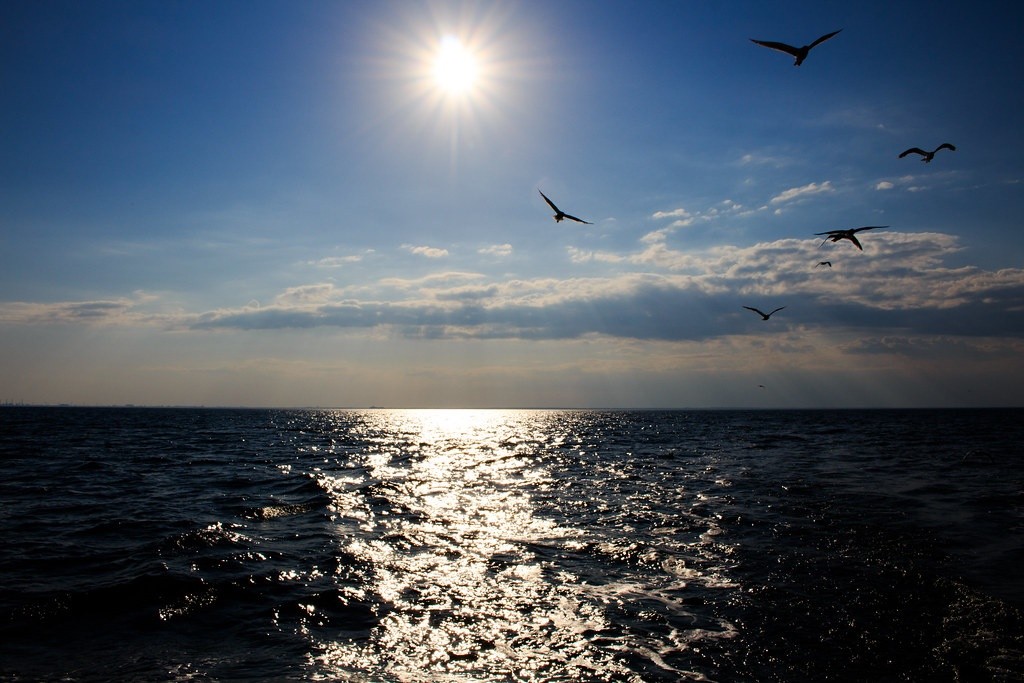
[
  {"xmin": 743, "ymin": 305, "xmax": 787, "ymax": 320},
  {"xmin": 812, "ymin": 225, "xmax": 892, "ymax": 267},
  {"xmin": 749, "ymin": 28, "xmax": 844, "ymax": 65},
  {"xmin": 899, "ymin": 143, "xmax": 957, "ymax": 164},
  {"xmin": 539, "ymin": 190, "xmax": 596, "ymax": 225}
]
